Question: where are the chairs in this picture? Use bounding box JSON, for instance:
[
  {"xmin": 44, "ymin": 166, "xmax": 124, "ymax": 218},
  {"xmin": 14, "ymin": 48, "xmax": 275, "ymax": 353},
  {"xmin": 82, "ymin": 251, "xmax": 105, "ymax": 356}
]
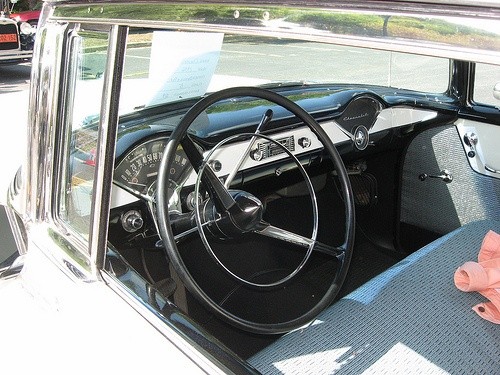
[{"xmin": 246, "ymin": 220, "xmax": 500, "ymax": 375}]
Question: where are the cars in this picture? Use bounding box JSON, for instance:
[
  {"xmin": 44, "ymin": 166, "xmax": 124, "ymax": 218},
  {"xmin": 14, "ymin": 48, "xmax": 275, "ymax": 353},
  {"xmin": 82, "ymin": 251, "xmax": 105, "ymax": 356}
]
[
  {"xmin": 0, "ymin": 9, "xmax": 45, "ymax": 66},
  {"xmin": 7, "ymin": 0, "xmax": 499, "ymax": 375}
]
